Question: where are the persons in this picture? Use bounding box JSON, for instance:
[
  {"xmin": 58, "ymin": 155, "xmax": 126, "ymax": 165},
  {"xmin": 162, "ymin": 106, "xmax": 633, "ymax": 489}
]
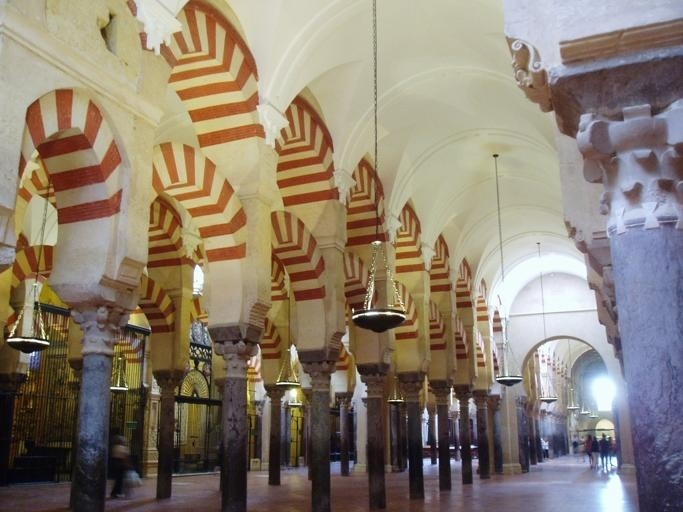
[
  {"xmin": 108, "ymin": 434, "xmax": 133, "ymax": 499},
  {"xmin": 539, "ymin": 430, "xmax": 619, "ymax": 473}
]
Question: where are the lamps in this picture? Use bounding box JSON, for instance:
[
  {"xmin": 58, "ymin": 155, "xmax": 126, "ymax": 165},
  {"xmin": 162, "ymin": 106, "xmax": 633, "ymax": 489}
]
[
  {"xmin": 108, "ymin": 329, "xmax": 405, "ymax": 412},
  {"xmin": 3, "ymin": 170, "xmax": 56, "ymax": 355},
  {"xmin": 486, "ymin": 152, "xmax": 602, "ymax": 421},
  {"xmin": 343, "ymin": 1, "xmax": 410, "ymax": 335}
]
